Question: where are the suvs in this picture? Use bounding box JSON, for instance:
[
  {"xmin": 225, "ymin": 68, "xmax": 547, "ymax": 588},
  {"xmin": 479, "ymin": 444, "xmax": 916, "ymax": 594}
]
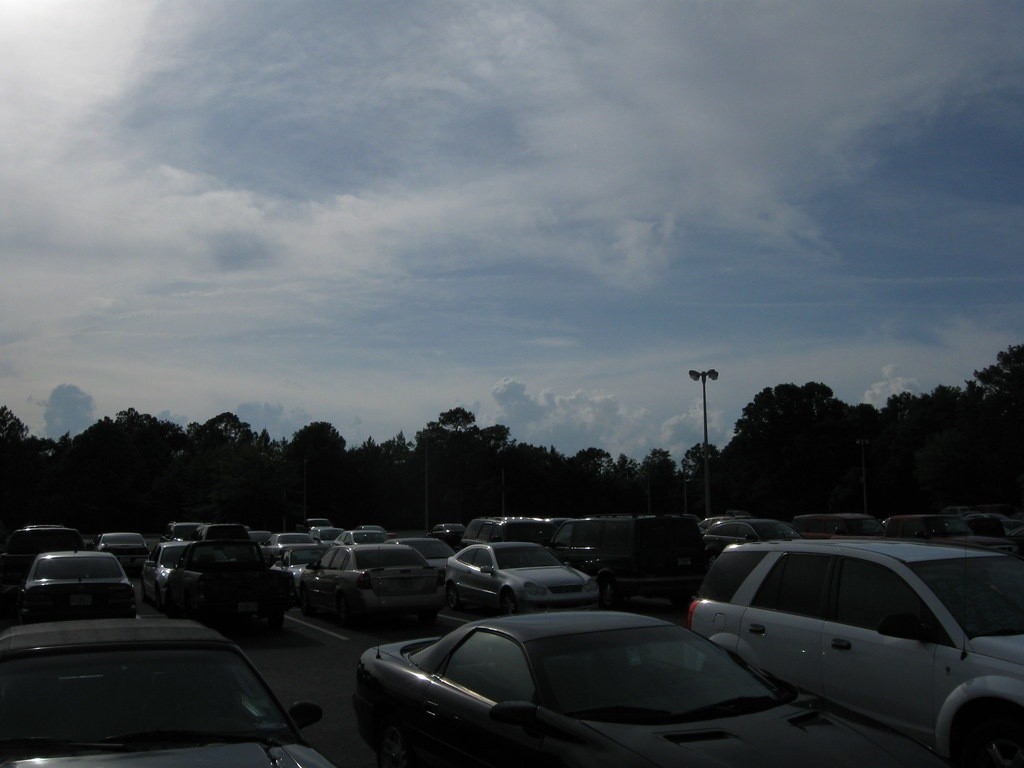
[
  {"xmin": 456, "ymin": 516, "xmax": 558, "ymax": 566},
  {"xmin": 688, "ymin": 536, "xmax": 1024, "ymax": 768},
  {"xmin": 541, "ymin": 512, "xmax": 709, "ymax": 610}
]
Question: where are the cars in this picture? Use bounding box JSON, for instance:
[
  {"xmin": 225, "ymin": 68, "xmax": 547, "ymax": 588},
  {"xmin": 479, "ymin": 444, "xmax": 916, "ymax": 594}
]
[
  {"xmin": 354, "ymin": 525, "xmax": 388, "ymax": 535},
  {"xmin": 354, "ymin": 611, "xmax": 966, "ymax": 768},
  {"xmin": 140, "ymin": 517, "xmax": 345, "ymax": 632},
  {"xmin": 384, "ymin": 538, "xmax": 457, "ymax": 584},
  {"xmin": 699, "ymin": 504, "xmax": 1024, "ymax": 579},
  {"xmin": 299, "ymin": 545, "xmax": 447, "ymax": 630},
  {"xmin": 426, "ymin": 523, "xmax": 467, "ymax": 546},
  {"xmin": 444, "ymin": 541, "xmax": 602, "ymax": 614},
  {"xmin": 0, "ymin": 618, "xmax": 341, "ymax": 768},
  {"xmin": 92, "ymin": 531, "xmax": 149, "ymax": 570},
  {"xmin": 543, "ymin": 517, "xmax": 575, "ymax": 531},
  {"xmin": 19, "ymin": 549, "xmax": 138, "ymax": 624},
  {"xmin": 331, "ymin": 530, "xmax": 390, "ymax": 546}
]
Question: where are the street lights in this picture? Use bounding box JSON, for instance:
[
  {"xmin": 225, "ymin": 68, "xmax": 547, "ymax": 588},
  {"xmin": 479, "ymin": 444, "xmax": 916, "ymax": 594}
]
[
  {"xmin": 689, "ymin": 369, "xmax": 719, "ymax": 517},
  {"xmin": 856, "ymin": 439, "xmax": 870, "ymax": 515},
  {"xmin": 303, "ymin": 458, "xmax": 308, "ymax": 522}
]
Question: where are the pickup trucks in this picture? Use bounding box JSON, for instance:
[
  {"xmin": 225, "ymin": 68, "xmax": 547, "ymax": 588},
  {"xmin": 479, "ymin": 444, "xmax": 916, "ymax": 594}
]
[{"xmin": 0, "ymin": 524, "xmax": 96, "ymax": 582}]
